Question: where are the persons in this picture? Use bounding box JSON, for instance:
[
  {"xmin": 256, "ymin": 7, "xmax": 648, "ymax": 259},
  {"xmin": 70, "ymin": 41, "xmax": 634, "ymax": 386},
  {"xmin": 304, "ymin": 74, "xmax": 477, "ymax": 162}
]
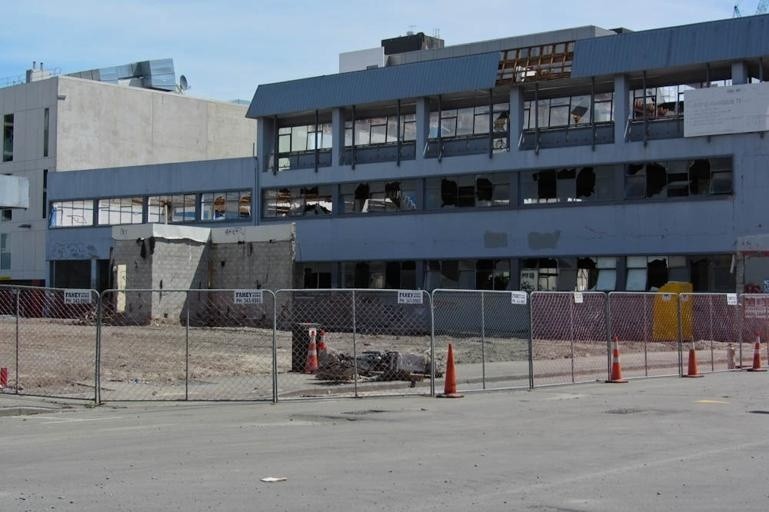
[{"xmin": 658, "ymin": 102, "xmax": 675, "ymax": 118}]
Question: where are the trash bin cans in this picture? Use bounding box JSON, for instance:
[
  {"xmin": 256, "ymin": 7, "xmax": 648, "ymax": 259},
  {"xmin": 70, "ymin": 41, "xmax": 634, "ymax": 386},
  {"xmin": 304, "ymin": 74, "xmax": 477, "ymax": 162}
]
[{"xmin": 292, "ymin": 323, "xmax": 321, "ymax": 372}]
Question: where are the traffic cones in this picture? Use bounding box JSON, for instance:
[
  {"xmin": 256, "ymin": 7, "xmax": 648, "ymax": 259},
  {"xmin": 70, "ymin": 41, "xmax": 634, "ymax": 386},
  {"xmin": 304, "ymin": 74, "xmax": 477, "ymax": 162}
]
[
  {"xmin": 437, "ymin": 342, "xmax": 464, "ymax": 399},
  {"xmin": 605, "ymin": 334, "xmax": 629, "ymax": 383},
  {"xmin": 746, "ymin": 331, "xmax": 768, "ymax": 372},
  {"xmin": 301, "ymin": 330, "xmax": 327, "ymax": 374},
  {"xmin": 684, "ymin": 334, "xmax": 704, "ymax": 379}
]
